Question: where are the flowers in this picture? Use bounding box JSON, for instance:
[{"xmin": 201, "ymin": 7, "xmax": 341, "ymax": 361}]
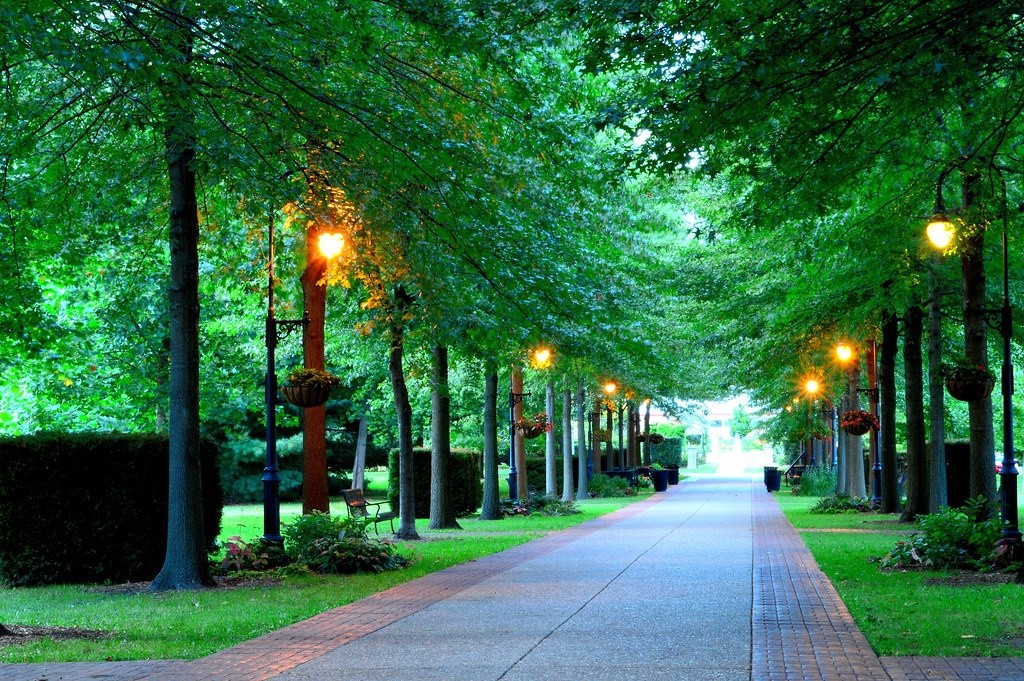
[
  {"xmin": 935, "ymin": 358, "xmax": 997, "ymax": 381},
  {"xmin": 513, "ymin": 413, "xmax": 554, "ymax": 431},
  {"xmin": 841, "ymin": 409, "xmax": 880, "ymax": 435}
]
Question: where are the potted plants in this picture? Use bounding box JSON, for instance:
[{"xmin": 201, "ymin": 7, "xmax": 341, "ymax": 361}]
[
  {"xmin": 592, "ymin": 429, "xmax": 612, "ymax": 442},
  {"xmin": 635, "ymin": 433, "xmax": 648, "ymax": 442},
  {"xmin": 648, "ymin": 433, "xmax": 664, "ymax": 444},
  {"xmin": 281, "ymin": 366, "xmax": 337, "ymax": 407},
  {"xmin": 812, "ymin": 427, "xmax": 834, "ymax": 440}
]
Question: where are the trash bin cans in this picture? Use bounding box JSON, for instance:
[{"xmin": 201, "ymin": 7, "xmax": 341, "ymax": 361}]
[
  {"xmin": 653, "ymin": 470, "xmax": 667, "ymax": 491},
  {"xmin": 766, "ymin": 470, "xmax": 781, "ymax": 491}
]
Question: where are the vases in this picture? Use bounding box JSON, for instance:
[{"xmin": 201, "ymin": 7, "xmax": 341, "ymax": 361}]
[
  {"xmin": 850, "ymin": 427, "xmax": 869, "ymax": 437},
  {"xmin": 945, "ymin": 379, "xmax": 995, "ymax": 402},
  {"xmin": 516, "ymin": 429, "xmax": 541, "ymax": 438}
]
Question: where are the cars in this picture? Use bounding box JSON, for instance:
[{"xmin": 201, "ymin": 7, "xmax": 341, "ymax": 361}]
[{"xmin": 994, "ymin": 455, "xmax": 1018, "ymax": 474}]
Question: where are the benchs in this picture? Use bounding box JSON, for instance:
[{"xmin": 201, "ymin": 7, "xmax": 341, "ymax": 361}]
[{"xmin": 339, "ymin": 489, "xmax": 397, "ymax": 535}]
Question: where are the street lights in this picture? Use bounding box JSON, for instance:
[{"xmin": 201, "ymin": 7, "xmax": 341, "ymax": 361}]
[
  {"xmin": 836, "ymin": 316, "xmax": 882, "ymax": 510},
  {"xmin": 510, "ymin": 334, "xmax": 550, "ymax": 497},
  {"xmin": 925, "ymin": 150, "xmax": 1023, "ymax": 566},
  {"xmin": 806, "ymin": 362, "xmax": 837, "ymax": 488},
  {"xmin": 263, "ymin": 166, "xmax": 348, "ymax": 564},
  {"xmin": 586, "ymin": 375, "xmax": 616, "ymax": 483}
]
